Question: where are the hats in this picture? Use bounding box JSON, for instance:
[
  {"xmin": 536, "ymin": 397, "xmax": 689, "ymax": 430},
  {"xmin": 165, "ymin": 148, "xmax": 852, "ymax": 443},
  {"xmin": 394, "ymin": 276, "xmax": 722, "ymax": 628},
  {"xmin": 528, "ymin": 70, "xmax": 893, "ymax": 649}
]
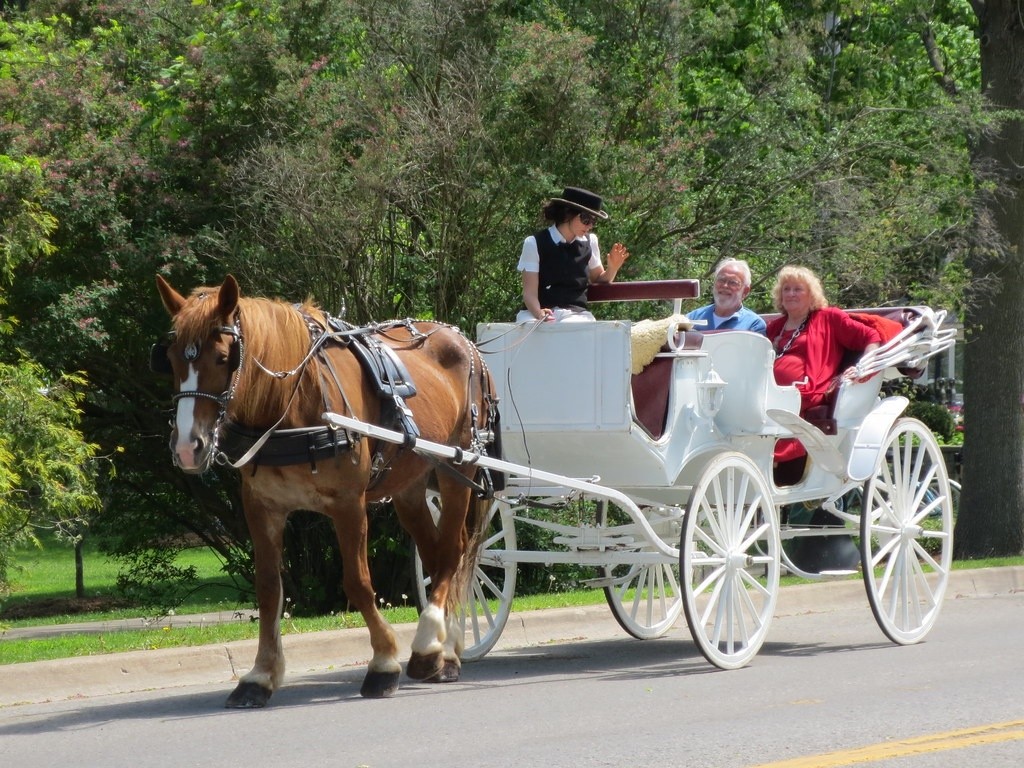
[{"xmin": 550, "ymin": 186, "xmax": 608, "ymax": 220}]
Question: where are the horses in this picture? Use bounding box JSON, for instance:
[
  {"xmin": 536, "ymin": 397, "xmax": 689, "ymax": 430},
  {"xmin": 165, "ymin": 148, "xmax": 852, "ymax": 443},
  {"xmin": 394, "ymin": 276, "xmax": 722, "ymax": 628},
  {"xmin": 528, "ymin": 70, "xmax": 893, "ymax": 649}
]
[{"xmin": 155, "ymin": 272, "xmax": 509, "ymax": 711}]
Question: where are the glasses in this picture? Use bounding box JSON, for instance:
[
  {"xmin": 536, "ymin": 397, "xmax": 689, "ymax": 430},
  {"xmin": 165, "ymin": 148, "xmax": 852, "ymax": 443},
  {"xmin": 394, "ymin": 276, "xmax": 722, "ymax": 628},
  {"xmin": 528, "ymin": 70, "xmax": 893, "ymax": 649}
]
[
  {"xmin": 579, "ymin": 212, "xmax": 603, "ymax": 229},
  {"xmin": 717, "ymin": 276, "xmax": 740, "ymax": 286}
]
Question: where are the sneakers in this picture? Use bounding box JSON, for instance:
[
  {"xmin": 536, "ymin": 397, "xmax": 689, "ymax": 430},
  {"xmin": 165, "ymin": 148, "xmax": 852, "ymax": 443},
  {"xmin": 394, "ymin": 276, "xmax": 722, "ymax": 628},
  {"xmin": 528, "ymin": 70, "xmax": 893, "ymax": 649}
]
[
  {"xmin": 747, "ymin": 507, "xmax": 763, "ymax": 528},
  {"xmin": 787, "ymin": 502, "xmax": 846, "ymax": 528}
]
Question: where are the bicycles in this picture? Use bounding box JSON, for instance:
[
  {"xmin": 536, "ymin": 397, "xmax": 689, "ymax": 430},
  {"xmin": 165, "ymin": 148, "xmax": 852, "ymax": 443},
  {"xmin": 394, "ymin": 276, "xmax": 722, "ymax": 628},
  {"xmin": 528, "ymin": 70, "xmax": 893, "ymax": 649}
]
[{"xmin": 834, "ymin": 451, "xmax": 962, "ymax": 532}]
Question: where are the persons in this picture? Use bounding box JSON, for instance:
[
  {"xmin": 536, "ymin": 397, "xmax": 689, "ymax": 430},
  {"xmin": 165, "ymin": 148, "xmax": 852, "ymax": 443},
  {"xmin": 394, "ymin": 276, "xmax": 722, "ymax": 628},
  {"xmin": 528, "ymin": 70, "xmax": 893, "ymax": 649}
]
[
  {"xmin": 515, "ymin": 186, "xmax": 630, "ymax": 325},
  {"xmin": 681, "ymin": 258, "xmax": 767, "ymax": 337},
  {"xmin": 761, "ymin": 265, "xmax": 882, "ymax": 486}
]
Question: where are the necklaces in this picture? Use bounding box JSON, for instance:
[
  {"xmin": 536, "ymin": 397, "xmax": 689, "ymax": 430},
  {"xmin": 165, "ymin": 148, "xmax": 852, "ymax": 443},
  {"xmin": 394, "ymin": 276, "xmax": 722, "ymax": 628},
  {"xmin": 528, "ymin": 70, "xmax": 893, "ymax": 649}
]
[{"xmin": 770, "ymin": 315, "xmax": 808, "ymax": 362}]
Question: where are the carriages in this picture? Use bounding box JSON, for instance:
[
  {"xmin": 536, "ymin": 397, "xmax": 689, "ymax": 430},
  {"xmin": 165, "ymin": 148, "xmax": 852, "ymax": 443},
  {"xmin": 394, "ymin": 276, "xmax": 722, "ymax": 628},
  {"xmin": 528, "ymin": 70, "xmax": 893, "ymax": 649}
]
[{"xmin": 151, "ymin": 274, "xmax": 958, "ymax": 708}]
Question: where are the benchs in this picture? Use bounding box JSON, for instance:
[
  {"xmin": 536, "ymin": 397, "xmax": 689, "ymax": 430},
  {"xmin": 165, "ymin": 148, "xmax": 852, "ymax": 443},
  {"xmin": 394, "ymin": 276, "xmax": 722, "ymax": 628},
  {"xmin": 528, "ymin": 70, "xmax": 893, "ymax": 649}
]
[
  {"xmin": 585, "ymin": 278, "xmax": 707, "ymax": 360},
  {"xmin": 695, "ymin": 327, "xmax": 803, "ymax": 433},
  {"xmin": 757, "ymin": 307, "xmax": 921, "ymax": 421}
]
